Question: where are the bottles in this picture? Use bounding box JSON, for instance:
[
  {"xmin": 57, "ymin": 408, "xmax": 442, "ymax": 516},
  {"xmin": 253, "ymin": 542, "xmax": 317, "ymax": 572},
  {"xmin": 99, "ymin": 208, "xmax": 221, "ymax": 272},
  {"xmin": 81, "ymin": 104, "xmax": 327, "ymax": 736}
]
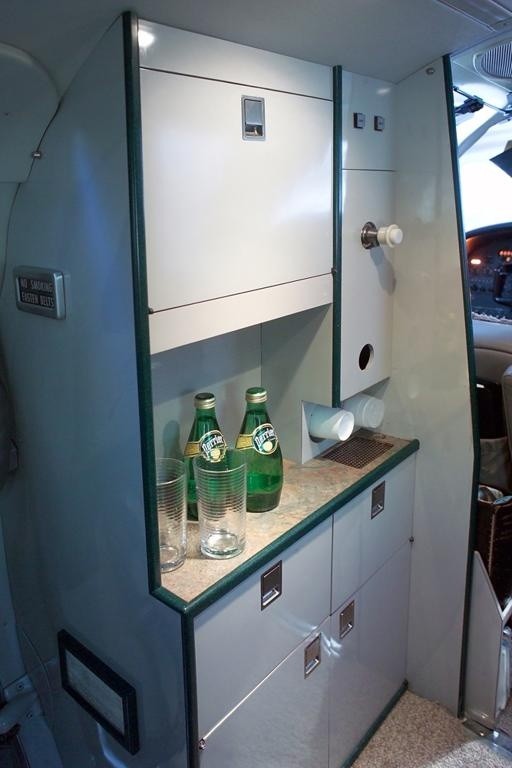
[
  {"xmin": 183, "ymin": 392, "xmax": 229, "ymax": 521},
  {"xmin": 234, "ymin": 386, "xmax": 283, "ymax": 512}
]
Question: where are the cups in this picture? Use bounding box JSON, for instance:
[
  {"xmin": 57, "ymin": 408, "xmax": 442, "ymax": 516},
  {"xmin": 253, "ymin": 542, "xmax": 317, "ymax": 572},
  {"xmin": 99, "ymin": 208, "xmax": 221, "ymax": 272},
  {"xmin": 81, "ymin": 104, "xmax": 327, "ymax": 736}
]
[
  {"xmin": 194, "ymin": 448, "xmax": 249, "ymax": 561},
  {"xmin": 154, "ymin": 458, "xmax": 188, "ymax": 575},
  {"xmin": 308, "ymin": 393, "xmax": 385, "ymax": 442}
]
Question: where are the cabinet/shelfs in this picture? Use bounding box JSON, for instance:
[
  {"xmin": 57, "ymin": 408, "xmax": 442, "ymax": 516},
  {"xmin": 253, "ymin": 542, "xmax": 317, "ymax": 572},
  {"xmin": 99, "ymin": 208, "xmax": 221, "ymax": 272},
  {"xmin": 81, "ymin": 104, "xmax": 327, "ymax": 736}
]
[{"xmin": 4, "ymin": 11, "xmax": 418, "ymax": 768}]
